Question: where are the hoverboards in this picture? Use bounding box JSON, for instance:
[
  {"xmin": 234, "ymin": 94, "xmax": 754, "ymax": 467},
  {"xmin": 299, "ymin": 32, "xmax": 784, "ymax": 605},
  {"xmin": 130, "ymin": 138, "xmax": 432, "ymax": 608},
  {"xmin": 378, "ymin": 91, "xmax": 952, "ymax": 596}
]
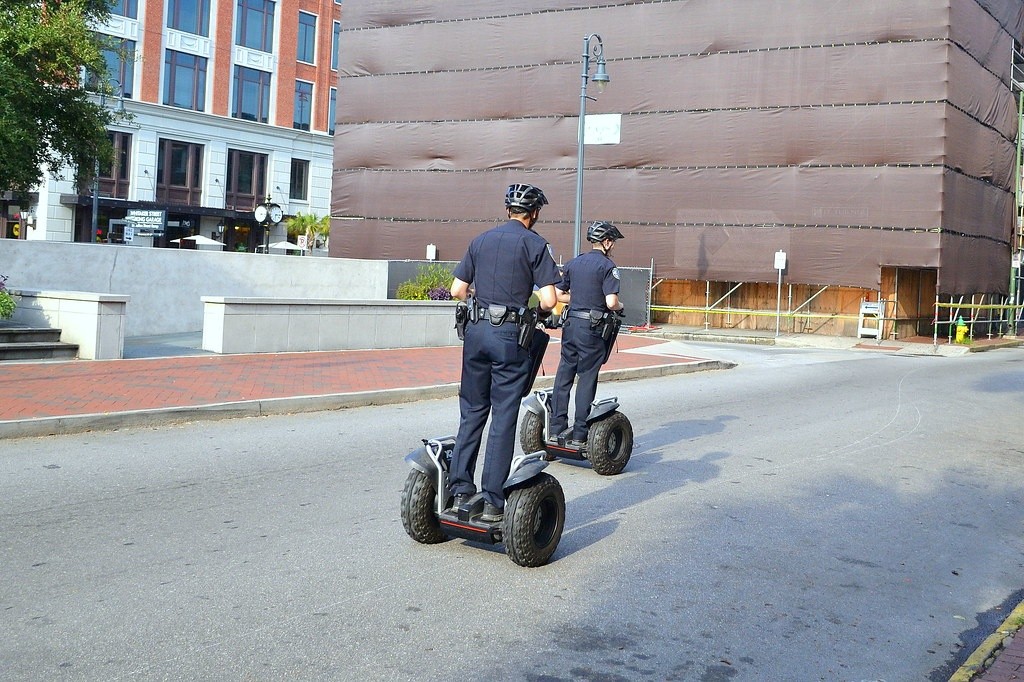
[
  {"xmin": 399, "ymin": 307, "xmax": 567, "ymax": 567},
  {"xmin": 520, "ymin": 307, "xmax": 636, "ymax": 474}
]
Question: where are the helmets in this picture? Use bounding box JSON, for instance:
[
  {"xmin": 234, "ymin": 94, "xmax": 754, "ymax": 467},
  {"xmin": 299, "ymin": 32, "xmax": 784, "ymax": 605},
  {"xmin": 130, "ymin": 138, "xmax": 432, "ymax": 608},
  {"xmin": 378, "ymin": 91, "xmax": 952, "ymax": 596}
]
[
  {"xmin": 586, "ymin": 221, "xmax": 625, "ymax": 242},
  {"xmin": 504, "ymin": 182, "xmax": 549, "ymax": 212}
]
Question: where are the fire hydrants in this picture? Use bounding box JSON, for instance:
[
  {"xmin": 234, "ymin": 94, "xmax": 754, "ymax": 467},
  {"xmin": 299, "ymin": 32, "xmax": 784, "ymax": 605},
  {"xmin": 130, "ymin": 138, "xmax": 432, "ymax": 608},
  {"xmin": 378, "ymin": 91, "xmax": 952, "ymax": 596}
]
[{"xmin": 955, "ymin": 315, "xmax": 969, "ymax": 341}]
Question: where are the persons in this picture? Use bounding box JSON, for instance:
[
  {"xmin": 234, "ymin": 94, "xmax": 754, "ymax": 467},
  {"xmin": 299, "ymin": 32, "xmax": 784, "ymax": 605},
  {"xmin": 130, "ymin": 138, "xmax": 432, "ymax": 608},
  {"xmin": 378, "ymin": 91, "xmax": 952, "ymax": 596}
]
[
  {"xmin": 550, "ymin": 222, "xmax": 623, "ymax": 447},
  {"xmin": 451, "ymin": 182, "xmax": 560, "ymax": 520}
]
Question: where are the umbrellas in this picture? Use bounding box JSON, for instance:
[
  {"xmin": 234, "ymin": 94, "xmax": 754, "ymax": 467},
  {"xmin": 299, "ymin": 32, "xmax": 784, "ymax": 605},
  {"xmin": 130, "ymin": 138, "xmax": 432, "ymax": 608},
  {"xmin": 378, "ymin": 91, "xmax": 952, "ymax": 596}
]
[
  {"xmin": 170, "ymin": 235, "xmax": 227, "ymax": 250},
  {"xmin": 258, "ymin": 241, "xmax": 306, "ymax": 255}
]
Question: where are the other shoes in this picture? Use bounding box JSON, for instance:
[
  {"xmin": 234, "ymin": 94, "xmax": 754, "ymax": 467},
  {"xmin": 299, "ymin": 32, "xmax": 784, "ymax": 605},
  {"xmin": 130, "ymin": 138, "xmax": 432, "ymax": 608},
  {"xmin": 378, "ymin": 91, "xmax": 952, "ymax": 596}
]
[
  {"xmin": 451, "ymin": 493, "xmax": 469, "ymax": 513},
  {"xmin": 572, "ymin": 438, "xmax": 584, "ymax": 447},
  {"xmin": 480, "ymin": 500, "xmax": 505, "ymax": 521},
  {"xmin": 549, "ymin": 434, "xmax": 558, "ymax": 442}
]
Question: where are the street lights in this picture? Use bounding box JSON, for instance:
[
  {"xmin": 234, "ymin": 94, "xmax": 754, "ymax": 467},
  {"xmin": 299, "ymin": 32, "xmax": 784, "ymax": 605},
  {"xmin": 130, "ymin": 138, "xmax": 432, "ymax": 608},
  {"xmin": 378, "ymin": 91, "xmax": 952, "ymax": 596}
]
[{"xmin": 574, "ymin": 31, "xmax": 608, "ymax": 266}]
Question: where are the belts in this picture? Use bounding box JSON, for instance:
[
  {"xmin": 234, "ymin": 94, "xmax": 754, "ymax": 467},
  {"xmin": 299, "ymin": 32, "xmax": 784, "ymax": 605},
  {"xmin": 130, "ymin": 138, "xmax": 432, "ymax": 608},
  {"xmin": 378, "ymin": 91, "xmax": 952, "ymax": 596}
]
[
  {"xmin": 567, "ymin": 310, "xmax": 591, "ymax": 321},
  {"xmin": 476, "ymin": 308, "xmax": 519, "ymax": 323}
]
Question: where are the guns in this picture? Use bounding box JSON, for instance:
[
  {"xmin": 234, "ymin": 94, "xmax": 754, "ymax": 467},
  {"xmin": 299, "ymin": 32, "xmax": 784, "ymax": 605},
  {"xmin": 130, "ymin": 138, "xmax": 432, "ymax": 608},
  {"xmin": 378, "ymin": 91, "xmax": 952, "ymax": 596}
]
[
  {"xmin": 602, "ymin": 312, "xmax": 614, "ymax": 325},
  {"xmin": 519, "ymin": 306, "xmax": 536, "ymax": 327}
]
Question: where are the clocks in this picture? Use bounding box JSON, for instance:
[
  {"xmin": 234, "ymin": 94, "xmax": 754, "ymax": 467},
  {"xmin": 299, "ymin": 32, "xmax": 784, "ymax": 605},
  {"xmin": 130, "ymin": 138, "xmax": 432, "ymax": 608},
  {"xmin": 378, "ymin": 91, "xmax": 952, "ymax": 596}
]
[
  {"xmin": 270, "ymin": 206, "xmax": 283, "ymax": 223},
  {"xmin": 254, "ymin": 205, "xmax": 267, "ymax": 223}
]
